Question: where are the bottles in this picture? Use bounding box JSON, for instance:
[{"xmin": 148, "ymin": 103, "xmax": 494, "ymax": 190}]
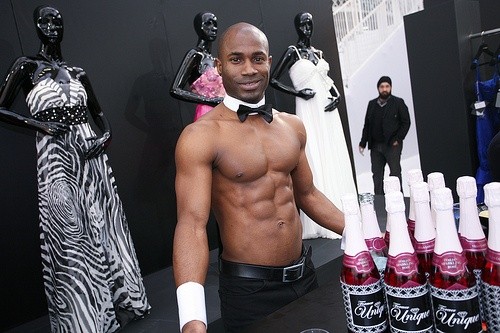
[{"xmin": 341, "ymin": 168, "xmax": 500, "ymax": 333}]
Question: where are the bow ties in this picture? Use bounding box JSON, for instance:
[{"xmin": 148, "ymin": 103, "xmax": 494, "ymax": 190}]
[{"xmin": 237, "ymin": 104, "xmax": 274, "ymax": 124}]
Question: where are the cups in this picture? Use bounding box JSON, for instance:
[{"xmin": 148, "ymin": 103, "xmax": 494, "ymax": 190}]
[{"xmin": 300, "ymin": 328, "xmax": 329, "ymax": 333}]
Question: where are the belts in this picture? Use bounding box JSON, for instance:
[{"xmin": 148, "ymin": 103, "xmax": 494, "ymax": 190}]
[{"xmin": 219, "ymin": 243, "xmax": 313, "ymax": 283}]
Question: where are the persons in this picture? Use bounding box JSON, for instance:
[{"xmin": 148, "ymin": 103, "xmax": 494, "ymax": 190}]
[
  {"xmin": 172, "ymin": 22, "xmax": 348, "ymax": 333},
  {"xmin": 359, "ymin": 77, "xmax": 412, "ymax": 194},
  {"xmin": 269, "ymin": 12, "xmax": 364, "ymax": 239},
  {"xmin": 0, "ymin": 5, "xmax": 153, "ymax": 333},
  {"xmin": 173, "ymin": 10, "xmax": 229, "ymax": 124}
]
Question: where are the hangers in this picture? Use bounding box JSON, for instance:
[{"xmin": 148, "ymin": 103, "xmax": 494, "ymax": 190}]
[
  {"xmin": 490, "ymin": 45, "xmax": 500, "ymax": 66},
  {"xmin": 471, "ymin": 31, "xmax": 493, "ymax": 69}
]
